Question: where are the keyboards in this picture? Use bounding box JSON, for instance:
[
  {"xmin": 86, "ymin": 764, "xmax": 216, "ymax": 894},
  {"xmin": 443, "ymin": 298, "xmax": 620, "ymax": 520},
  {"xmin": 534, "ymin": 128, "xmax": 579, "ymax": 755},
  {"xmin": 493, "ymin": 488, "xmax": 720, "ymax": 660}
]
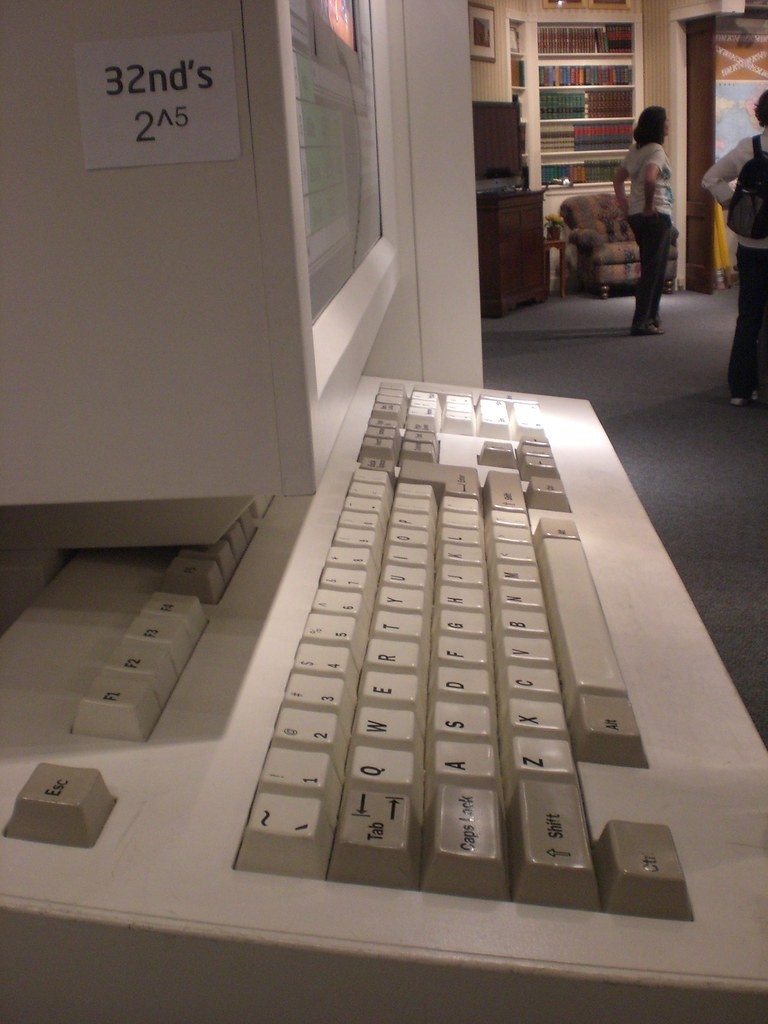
[{"xmin": 0, "ymin": 372, "xmax": 768, "ymax": 1024}]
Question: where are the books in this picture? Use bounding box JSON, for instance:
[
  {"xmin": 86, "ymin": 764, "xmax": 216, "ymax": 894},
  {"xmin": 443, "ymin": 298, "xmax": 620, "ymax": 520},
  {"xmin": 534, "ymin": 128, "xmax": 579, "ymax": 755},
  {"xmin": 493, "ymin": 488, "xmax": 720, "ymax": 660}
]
[{"xmin": 511, "ymin": 23, "xmax": 634, "ymax": 186}]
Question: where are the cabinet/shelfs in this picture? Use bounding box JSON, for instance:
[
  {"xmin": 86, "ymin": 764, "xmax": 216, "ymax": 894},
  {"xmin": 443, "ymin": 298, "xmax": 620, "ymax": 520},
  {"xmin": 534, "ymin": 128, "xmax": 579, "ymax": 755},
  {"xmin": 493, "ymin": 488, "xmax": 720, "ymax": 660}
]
[
  {"xmin": 476, "ymin": 189, "xmax": 548, "ymax": 318},
  {"xmin": 506, "ymin": 9, "xmax": 644, "ymax": 191}
]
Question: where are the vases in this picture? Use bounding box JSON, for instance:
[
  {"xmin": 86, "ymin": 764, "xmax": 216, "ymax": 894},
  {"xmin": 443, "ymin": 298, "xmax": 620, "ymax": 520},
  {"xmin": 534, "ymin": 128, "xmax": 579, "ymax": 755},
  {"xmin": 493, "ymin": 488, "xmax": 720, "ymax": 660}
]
[{"xmin": 547, "ymin": 226, "xmax": 561, "ymax": 241}]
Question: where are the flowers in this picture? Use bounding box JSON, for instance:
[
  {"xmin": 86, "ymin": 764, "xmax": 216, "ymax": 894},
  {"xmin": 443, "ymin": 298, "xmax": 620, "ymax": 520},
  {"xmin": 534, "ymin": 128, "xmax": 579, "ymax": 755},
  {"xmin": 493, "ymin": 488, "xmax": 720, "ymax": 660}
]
[{"xmin": 545, "ymin": 214, "xmax": 565, "ymax": 230}]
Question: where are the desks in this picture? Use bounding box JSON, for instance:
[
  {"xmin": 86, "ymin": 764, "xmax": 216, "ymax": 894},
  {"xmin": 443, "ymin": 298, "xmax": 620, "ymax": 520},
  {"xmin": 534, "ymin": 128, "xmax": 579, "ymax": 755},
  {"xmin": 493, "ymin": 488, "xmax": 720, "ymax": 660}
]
[{"xmin": 543, "ymin": 240, "xmax": 565, "ymax": 297}]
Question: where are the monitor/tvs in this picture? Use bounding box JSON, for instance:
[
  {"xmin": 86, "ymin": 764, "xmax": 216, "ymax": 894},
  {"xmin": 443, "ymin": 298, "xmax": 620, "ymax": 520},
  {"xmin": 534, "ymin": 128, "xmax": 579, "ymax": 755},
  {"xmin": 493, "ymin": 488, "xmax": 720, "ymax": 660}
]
[{"xmin": 0, "ymin": 0, "xmax": 398, "ymax": 627}]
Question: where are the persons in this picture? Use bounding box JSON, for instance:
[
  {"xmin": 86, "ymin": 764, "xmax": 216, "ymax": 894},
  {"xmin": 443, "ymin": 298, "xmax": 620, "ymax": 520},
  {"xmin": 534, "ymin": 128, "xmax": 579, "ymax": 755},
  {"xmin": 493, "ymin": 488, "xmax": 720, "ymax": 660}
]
[
  {"xmin": 702, "ymin": 89, "xmax": 768, "ymax": 407},
  {"xmin": 614, "ymin": 106, "xmax": 672, "ymax": 335}
]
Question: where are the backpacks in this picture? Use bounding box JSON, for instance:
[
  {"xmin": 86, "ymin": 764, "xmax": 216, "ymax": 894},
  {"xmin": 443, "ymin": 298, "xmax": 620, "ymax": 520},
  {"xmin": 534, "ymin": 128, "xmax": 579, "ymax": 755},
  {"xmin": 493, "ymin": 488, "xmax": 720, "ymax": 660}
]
[{"xmin": 727, "ymin": 135, "xmax": 768, "ymax": 239}]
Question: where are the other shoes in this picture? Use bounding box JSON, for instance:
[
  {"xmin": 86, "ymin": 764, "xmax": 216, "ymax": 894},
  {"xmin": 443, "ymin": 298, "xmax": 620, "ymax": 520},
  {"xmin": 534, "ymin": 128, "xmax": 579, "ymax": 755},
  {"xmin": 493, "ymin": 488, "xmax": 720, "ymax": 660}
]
[
  {"xmin": 651, "ymin": 318, "xmax": 660, "ymax": 326},
  {"xmin": 631, "ymin": 323, "xmax": 664, "ymax": 336},
  {"xmin": 730, "ymin": 390, "xmax": 758, "ymax": 406}
]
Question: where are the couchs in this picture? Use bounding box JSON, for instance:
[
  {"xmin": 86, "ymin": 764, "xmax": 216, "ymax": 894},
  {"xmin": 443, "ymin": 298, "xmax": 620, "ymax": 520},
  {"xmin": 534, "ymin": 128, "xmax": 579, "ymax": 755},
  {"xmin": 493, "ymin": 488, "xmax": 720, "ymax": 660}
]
[{"xmin": 560, "ymin": 194, "xmax": 679, "ymax": 300}]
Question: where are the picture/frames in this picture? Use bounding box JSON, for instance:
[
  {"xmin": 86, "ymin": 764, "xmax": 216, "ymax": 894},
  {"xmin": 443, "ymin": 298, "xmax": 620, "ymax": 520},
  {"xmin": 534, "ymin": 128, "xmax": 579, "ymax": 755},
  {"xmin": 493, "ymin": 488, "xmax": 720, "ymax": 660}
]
[{"xmin": 468, "ymin": 1, "xmax": 496, "ymax": 63}]
